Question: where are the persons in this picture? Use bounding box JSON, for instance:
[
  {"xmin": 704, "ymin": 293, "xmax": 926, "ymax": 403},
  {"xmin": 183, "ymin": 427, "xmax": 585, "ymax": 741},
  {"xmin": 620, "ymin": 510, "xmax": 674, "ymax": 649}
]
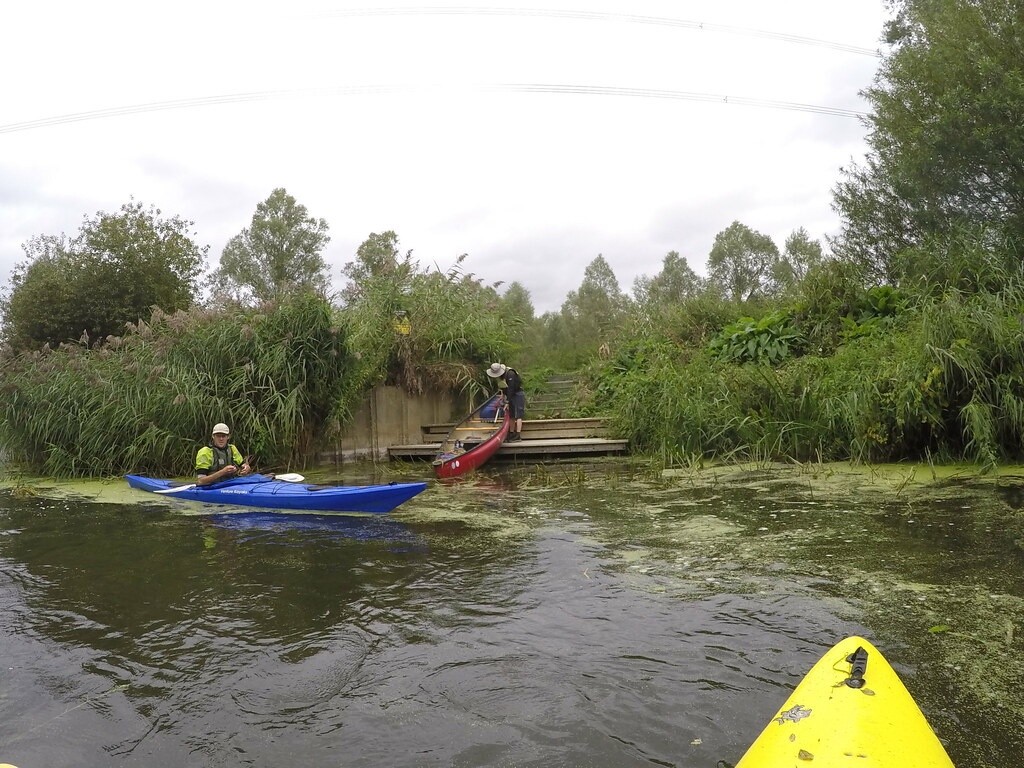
[
  {"xmin": 195, "ymin": 423, "xmax": 251, "ymax": 486},
  {"xmin": 486, "ymin": 362, "xmax": 525, "ymax": 443}
]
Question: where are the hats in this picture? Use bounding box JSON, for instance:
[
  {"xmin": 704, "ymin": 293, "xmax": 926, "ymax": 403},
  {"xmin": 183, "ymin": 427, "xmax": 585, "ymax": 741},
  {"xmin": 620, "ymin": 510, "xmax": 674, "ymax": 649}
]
[
  {"xmin": 486, "ymin": 362, "xmax": 506, "ymax": 378},
  {"xmin": 212, "ymin": 423, "xmax": 230, "ymax": 434}
]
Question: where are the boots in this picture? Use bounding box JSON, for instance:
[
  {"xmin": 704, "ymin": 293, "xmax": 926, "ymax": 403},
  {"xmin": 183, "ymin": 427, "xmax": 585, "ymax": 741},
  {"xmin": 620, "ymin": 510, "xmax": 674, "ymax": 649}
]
[
  {"xmin": 504, "ymin": 432, "xmax": 515, "ymax": 443},
  {"xmin": 509, "ymin": 432, "xmax": 521, "ymax": 442}
]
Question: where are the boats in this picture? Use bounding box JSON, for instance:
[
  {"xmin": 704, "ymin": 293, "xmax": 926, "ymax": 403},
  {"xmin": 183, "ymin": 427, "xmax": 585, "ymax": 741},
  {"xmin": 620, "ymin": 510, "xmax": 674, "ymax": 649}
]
[
  {"xmin": 125, "ymin": 473, "xmax": 429, "ymax": 517},
  {"xmin": 432, "ymin": 390, "xmax": 510, "ymax": 479},
  {"xmin": 715, "ymin": 635, "xmax": 960, "ymax": 768}
]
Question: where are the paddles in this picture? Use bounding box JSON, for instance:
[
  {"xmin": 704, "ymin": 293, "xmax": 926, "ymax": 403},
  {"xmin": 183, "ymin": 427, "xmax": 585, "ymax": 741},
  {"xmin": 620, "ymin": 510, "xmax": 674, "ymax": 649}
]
[{"xmin": 151, "ymin": 472, "xmax": 306, "ymax": 495}]
